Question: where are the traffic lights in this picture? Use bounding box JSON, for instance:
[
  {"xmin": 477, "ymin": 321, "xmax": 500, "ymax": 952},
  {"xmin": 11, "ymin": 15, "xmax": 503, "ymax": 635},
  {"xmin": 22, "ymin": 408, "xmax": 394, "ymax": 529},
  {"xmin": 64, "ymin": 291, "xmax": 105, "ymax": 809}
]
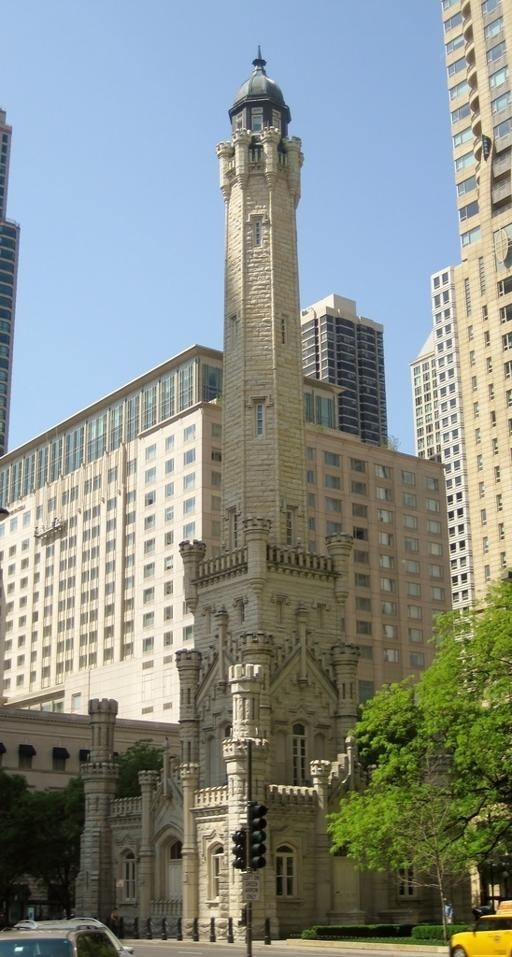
[
  {"xmin": 232, "ymin": 831, "xmax": 247, "ymax": 868},
  {"xmin": 249, "ymin": 802, "xmax": 267, "ymax": 869}
]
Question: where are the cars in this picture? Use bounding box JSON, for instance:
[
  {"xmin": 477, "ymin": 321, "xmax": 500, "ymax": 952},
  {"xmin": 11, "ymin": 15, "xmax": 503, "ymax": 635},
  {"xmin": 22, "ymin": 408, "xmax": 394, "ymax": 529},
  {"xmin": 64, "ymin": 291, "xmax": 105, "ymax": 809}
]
[
  {"xmin": 448, "ymin": 900, "xmax": 512, "ymax": 957},
  {"xmin": 0, "ymin": 916, "xmax": 136, "ymax": 957}
]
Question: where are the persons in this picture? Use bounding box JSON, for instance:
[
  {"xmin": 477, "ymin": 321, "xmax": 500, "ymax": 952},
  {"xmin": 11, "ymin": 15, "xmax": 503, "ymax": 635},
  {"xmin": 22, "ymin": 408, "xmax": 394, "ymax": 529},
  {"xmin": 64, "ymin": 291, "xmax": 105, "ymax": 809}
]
[
  {"xmin": 0, "ymin": 913, "xmax": 13, "ymax": 931},
  {"xmin": 443, "ymin": 898, "xmax": 455, "ymax": 924},
  {"xmin": 59, "ymin": 903, "xmax": 68, "ymax": 921},
  {"xmin": 105, "ymin": 908, "xmax": 120, "ymax": 934}
]
